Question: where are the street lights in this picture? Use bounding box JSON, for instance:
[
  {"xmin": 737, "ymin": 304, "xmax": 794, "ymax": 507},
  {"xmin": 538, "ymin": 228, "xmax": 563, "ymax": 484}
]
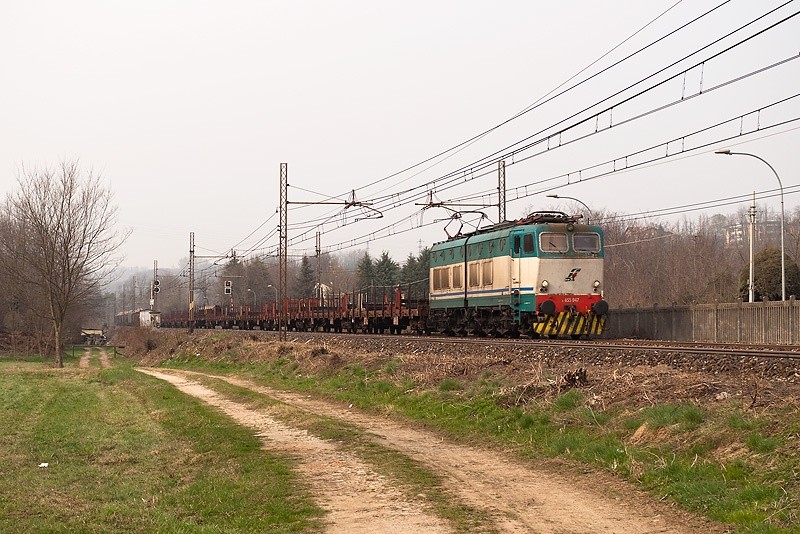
[
  {"xmin": 715, "ymin": 148, "xmax": 786, "ymax": 301},
  {"xmin": 267, "ymin": 284, "xmax": 277, "ymax": 309},
  {"xmin": 546, "ymin": 195, "xmax": 592, "ymax": 215},
  {"xmin": 248, "ymin": 288, "xmax": 256, "ymax": 312}
]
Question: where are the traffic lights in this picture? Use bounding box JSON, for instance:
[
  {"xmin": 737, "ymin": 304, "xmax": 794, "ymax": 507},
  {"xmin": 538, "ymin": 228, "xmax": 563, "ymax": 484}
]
[{"xmin": 225, "ymin": 281, "xmax": 231, "ymax": 295}]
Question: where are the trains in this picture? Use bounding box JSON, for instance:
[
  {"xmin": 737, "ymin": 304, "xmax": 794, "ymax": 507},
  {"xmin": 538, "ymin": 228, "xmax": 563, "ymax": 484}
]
[{"xmin": 160, "ymin": 210, "xmax": 610, "ymax": 340}]
